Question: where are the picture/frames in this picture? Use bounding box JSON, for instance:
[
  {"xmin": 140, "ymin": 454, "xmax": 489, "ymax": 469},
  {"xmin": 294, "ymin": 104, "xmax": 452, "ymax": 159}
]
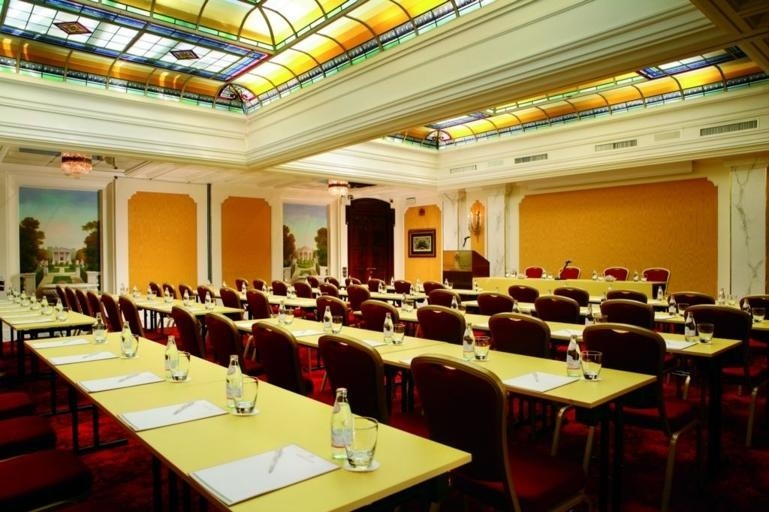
[
  {"xmin": 4, "ymin": 169, "xmax": 117, "ymax": 298},
  {"xmin": 409, "ymin": 230, "xmax": 436, "ymax": 257}
]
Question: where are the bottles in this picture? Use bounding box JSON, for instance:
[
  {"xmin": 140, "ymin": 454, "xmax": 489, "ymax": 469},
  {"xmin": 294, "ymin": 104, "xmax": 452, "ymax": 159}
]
[
  {"xmin": 165, "ymin": 334, "xmax": 179, "ymax": 378},
  {"xmin": 382, "ymin": 313, "xmax": 395, "ymax": 343},
  {"xmin": 120, "ymin": 321, "xmax": 131, "ymax": 358},
  {"xmin": 566, "ymin": 334, "xmax": 581, "ymax": 378},
  {"xmin": 682, "ymin": 311, "xmax": 698, "ymax": 343},
  {"xmin": 461, "ymin": 322, "xmax": 476, "ymax": 362},
  {"xmin": 330, "ymin": 387, "xmax": 354, "ymax": 458},
  {"xmin": 322, "ymin": 306, "xmax": 334, "ymax": 333},
  {"xmin": 226, "ymin": 355, "xmax": 243, "ymax": 411},
  {"xmin": 2, "ymin": 264, "xmax": 769, "ymax": 324},
  {"xmin": 94, "ymin": 312, "xmax": 106, "ymax": 341}
]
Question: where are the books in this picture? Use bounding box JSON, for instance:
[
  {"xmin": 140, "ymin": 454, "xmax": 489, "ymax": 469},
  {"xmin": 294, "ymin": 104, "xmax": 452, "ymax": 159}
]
[
  {"xmin": 664, "ymin": 337, "xmax": 697, "ymax": 350},
  {"xmin": 30, "ymin": 337, "xmax": 91, "ymax": 348},
  {"xmin": 77, "ymin": 372, "xmax": 167, "ymax": 392},
  {"xmin": 504, "ymin": 372, "xmax": 580, "ymax": 392},
  {"xmin": 291, "ymin": 329, "xmax": 325, "ymax": 339},
  {"xmin": 551, "ymin": 328, "xmax": 583, "ymax": 338},
  {"xmin": 188, "ymin": 442, "xmax": 342, "ymax": 507},
  {"xmin": 117, "ymin": 401, "xmax": 229, "ymax": 431},
  {"xmin": 362, "ymin": 339, "xmax": 387, "ymax": 347},
  {"xmin": 0, "ymin": 299, "xmax": 55, "ymax": 326},
  {"xmin": 48, "ymin": 351, "xmax": 120, "ymax": 366}
]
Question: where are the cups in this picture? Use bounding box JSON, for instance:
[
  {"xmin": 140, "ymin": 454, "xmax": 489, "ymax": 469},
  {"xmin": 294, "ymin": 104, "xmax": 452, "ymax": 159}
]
[
  {"xmin": 581, "ymin": 351, "xmax": 604, "ymax": 380},
  {"xmin": 331, "ymin": 316, "xmax": 344, "ymax": 335},
  {"xmin": 474, "ymin": 336, "xmax": 491, "ymax": 361},
  {"xmin": 167, "ymin": 351, "xmax": 191, "ymax": 382},
  {"xmin": 121, "ymin": 334, "xmax": 139, "ymax": 357},
  {"xmin": 342, "ymin": 416, "xmax": 379, "ymax": 469},
  {"xmin": 393, "ymin": 323, "xmax": 407, "ymax": 344},
  {"xmin": 231, "ymin": 377, "xmax": 258, "ymax": 414},
  {"xmin": 698, "ymin": 323, "xmax": 715, "ymax": 344},
  {"xmin": 91, "ymin": 323, "xmax": 110, "ymax": 344}
]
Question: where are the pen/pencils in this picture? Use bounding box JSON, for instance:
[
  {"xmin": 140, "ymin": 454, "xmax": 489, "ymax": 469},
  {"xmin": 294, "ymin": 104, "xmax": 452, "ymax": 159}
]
[
  {"xmin": 174, "ymin": 401, "xmax": 195, "ymax": 414},
  {"xmin": 118, "ymin": 374, "xmax": 137, "ymax": 383},
  {"xmin": 268, "ymin": 448, "xmax": 282, "ymax": 474},
  {"xmin": 81, "ymin": 352, "xmax": 98, "ymax": 358},
  {"xmin": 534, "ymin": 372, "xmax": 539, "ymax": 383}
]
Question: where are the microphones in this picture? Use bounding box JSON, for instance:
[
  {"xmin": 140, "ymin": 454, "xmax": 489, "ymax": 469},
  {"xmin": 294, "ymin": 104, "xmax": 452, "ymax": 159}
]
[
  {"xmin": 555, "ymin": 260, "xmax": 572, "ymax": 280},
  {"xmin": 464, "ymin": 236, "xmax": 473, "ymax": 240}
]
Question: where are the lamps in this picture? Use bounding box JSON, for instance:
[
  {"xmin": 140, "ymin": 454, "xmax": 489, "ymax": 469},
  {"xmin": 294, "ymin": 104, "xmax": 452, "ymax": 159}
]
[
  {"xmin": 61, "ymin": 150, "xmax": 94, "ymax": 176},
  {"xmin": 328, "ymin": 178, "xmax": 348, "ymax": 197}
]
